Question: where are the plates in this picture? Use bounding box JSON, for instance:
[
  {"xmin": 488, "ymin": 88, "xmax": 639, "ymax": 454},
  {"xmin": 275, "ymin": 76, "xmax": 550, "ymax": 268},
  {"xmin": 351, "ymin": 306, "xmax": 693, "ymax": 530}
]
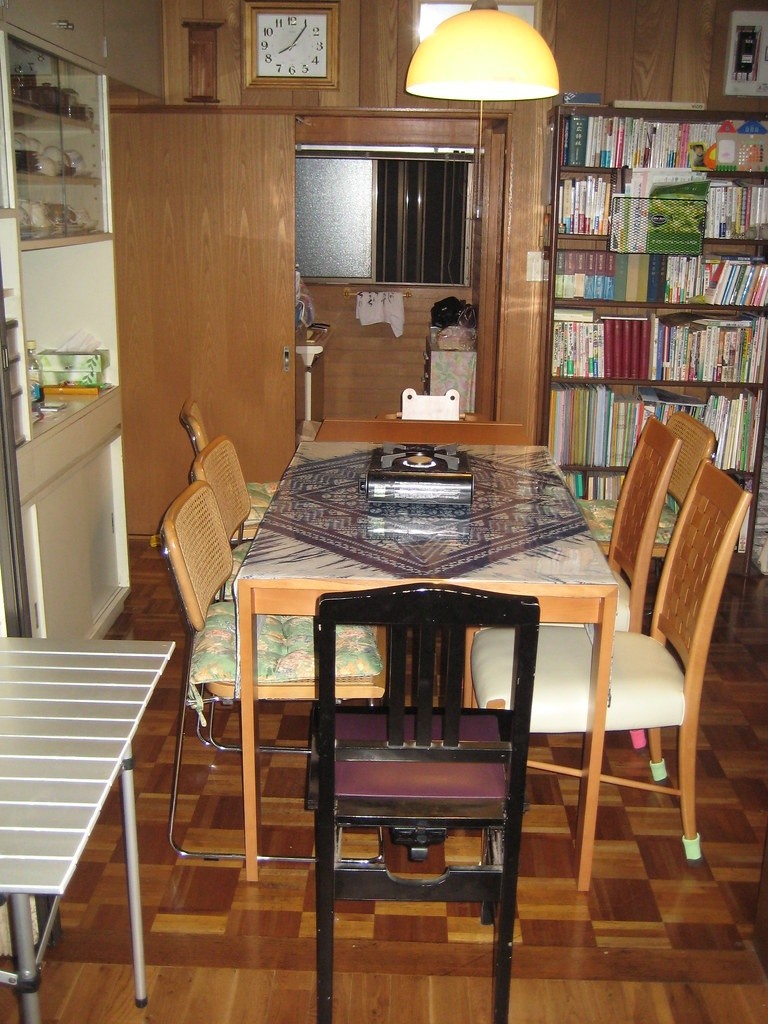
[{"xmin": 20, "ymin": 224, "xmax": 95, "ymax": 239}]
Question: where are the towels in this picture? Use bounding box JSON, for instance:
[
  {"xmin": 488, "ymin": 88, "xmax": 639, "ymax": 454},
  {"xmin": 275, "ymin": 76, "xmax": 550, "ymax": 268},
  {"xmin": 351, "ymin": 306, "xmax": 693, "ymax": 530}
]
[{"xmin": 355, "ymin": 290, "xmax": 405, "ymax": 338}]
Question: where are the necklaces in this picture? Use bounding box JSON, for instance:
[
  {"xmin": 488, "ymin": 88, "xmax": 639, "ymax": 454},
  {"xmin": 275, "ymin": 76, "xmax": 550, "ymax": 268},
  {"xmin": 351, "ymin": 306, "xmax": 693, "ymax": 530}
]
[{"xmin": 697, "ymin": 154, "xmax": 703, "ymax": 157}]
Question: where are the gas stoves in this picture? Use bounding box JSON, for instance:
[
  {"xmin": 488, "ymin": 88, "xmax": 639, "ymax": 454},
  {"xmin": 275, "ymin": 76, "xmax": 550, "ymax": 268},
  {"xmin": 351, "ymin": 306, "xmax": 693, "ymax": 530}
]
[{"xmin": 359, "ymin": 441, "xmax": 474, "ymax": 506}]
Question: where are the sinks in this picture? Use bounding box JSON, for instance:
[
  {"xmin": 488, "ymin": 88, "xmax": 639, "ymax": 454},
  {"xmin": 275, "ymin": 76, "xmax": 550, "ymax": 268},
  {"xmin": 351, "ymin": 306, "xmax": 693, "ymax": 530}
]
[{"xmin": 306, "ymin": 326, "xmax": 325, "ymax": 344}]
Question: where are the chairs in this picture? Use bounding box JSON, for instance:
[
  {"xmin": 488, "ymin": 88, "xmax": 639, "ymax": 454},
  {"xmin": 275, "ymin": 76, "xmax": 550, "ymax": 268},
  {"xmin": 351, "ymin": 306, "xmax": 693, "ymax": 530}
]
[
  {"xmin": 583, "ymin": 411, "xmax": 714, "ymax": 559},
  {"xmin": 468, "ymin": 460, "xmax": 751, "ymax": 860},
  {"xmin": 463, "ymin": 416, "xmax": 682, "ymax": 750},
  {"xmin": 307, "ymin": 580, "xmax": 538, "ymax": 1024},
  {"xmin": 189, "ymin": 435, "xmax": 312, "ymax": 751},
  {"xmin": 160, "ymin": 480, "xmax": 386, "ymax": 861},
  {"xmin": 179, "ymin": 397, "xmax": 273, "ymax": 517}
]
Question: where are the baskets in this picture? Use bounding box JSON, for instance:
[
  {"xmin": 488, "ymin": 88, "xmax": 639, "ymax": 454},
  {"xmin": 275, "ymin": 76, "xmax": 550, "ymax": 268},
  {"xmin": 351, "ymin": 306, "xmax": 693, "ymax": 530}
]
[{"xmin": 609, "ymin": 197, "xmax": 708, "ymax": 257}]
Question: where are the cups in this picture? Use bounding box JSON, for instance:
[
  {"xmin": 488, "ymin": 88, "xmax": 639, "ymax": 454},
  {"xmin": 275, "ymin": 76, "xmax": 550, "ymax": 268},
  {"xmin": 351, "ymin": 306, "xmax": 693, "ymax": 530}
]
[{"xmin": 17, "ymin": 196, "xmax": 96, "ymax": 227}]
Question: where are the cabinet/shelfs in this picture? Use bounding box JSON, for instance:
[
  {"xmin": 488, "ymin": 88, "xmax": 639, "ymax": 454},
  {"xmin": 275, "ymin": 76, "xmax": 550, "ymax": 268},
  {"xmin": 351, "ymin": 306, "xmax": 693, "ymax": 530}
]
[
  {"xmin": 0, "ymin": 0, "xmax": 166, "ymax": 249},
  {"xmin": 26, "ymin": 446, "xmax": 119, "ymax": 640},
  {"xmin": 538, "ymin": 106, "xmax": 767, "ymax": 579}
]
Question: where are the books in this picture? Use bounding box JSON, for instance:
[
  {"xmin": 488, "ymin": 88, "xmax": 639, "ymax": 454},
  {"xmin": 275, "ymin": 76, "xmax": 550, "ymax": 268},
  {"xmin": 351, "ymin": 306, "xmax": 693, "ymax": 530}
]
[
  {"xmin": 560, "ymin": 470, "xmax": 753, "ymax": 554},
  {"xmin": 560, "ymin": 114, "xmax": 768, "ymax": 172},
  {"xmin": 704, "ymin": 179, "xmax": 768, "ymax": 239},
  {"xmin": 551, "ymin": 309, "xmax": 768, "ymax": 383},
  {"xmin": 557, "ymin": 175, "xmax": 618, "ymax": 236},
  {"xmin": 554, "ymin": 250, "xmax": 768, "ymax": 307},
  {"xmin": 547, "ymin": 384, "xmax": 764, "ymax": 473}
]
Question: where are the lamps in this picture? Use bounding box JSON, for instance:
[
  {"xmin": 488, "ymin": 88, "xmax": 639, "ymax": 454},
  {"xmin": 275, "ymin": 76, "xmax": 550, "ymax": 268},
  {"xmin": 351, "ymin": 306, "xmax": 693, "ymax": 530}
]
[{"xmin": 404, "ymin": 0, "xmax": 560, "ymax": 102}]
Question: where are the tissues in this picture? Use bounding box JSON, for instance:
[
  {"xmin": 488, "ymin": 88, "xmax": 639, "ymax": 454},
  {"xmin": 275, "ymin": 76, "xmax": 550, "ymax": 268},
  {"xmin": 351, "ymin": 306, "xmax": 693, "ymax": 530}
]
[{"xmin": 35, "ymin": 327, "xmax": 111, "ymax": 386}]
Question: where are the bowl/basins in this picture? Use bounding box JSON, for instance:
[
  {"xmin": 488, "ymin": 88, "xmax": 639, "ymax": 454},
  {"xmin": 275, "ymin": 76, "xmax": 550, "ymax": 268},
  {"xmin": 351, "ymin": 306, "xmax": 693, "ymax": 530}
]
[{"xmin": 42, "ymin": 145, "xmax": 83, "ymax": 177}]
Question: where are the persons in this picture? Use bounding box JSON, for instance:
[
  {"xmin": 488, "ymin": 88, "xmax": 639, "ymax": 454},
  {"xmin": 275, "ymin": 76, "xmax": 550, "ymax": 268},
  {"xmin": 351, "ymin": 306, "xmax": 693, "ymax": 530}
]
[{"xmin": 693, "ymin": 145, "xmax": 708, "ymax": 168}]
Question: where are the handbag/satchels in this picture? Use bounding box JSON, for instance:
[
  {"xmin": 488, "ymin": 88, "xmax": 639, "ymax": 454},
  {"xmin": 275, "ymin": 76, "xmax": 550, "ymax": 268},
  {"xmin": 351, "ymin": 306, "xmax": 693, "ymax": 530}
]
[{"xmin": 430, "ymin": 296, "xmax": 476, "ymax": 329}]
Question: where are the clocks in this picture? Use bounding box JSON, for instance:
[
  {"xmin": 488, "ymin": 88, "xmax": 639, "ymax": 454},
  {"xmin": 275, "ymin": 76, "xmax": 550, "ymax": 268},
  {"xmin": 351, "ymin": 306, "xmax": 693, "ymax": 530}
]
[{"xmin": 240, "ymin": 1, "xmax": 339, "ymax": 90}]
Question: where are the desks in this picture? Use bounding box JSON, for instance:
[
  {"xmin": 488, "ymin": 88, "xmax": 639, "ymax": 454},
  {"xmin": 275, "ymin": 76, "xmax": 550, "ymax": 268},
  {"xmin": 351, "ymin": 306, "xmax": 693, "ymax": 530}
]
[
  {"xmin": 218, "ymin": 413, "xmax": 576, "ymax": 856},
  {"xmin": 0, "ymin": 637, "xmax": 177, "ymax": 1024}
]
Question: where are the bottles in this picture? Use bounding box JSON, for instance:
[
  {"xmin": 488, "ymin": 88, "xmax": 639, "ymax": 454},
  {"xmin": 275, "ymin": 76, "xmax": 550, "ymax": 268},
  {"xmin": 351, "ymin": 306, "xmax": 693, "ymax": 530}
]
[
  {"xmin": 14, "ymin": 132, "xmax": 41, "ymax": 177},
  {"xmin": 12, "ymin": 65, "xmax": 94, "ymax": 122},
  {"xmin": 27, "ymin": 341, "xmax": 45, "ymax": 402}
]
